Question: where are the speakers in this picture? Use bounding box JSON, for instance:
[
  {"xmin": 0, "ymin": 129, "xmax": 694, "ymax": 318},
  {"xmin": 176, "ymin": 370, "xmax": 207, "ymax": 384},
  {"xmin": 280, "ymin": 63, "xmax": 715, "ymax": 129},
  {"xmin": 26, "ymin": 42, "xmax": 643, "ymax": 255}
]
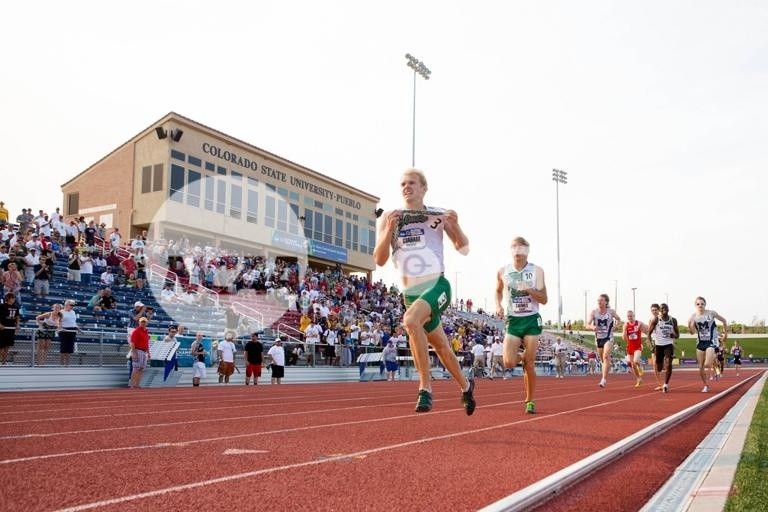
[
  {"xmin": 171, "ymin": 129, "xmax": 182, "ymax": 141},
  {"xmin": 155, "ymin": 126, "xmax": 166, "ymax": 138}
]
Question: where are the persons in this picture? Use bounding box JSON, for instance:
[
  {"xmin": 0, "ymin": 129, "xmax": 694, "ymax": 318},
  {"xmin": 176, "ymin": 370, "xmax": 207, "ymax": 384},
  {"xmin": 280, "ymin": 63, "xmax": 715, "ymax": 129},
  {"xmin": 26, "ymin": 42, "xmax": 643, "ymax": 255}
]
[
  {"xmin": 1, "ymin": 199, "xmax": 508, "ymax": 396},
  {"xmin": 373, "ymin": 167, "xmax": 478, "ymax": 418},
  {"xmin": 494, "ymin": 235, "xmax": 549, "ymax": 415},
  {"xmin": 509, "ymin": 290, "xmax": 751, "ymax": 397}
]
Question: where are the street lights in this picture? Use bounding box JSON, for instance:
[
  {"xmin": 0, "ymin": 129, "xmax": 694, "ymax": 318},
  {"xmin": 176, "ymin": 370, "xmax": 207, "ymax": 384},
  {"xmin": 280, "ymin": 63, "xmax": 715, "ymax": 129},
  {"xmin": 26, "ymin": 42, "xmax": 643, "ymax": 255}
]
[
  {"xmin": 551, "ymin": 169, "xmax": 567, "ymax": 319},
  {"xmin": 584, "ymin": 289, "xmax": 591, "ymax": 325},
  {"xmin": 631, "ymin": 288, "xmax": 637, "ymax": 314},
  {"xmin": 664, "ymin": 292, "xmax": 669, "ymax": 306},
  {"xmin": 614, "ymin": 280, "xmax": 618, "ymax": 311},
  {"xmin": 404, "ymin": 54, "xmax": 431, "ymax": 169}
]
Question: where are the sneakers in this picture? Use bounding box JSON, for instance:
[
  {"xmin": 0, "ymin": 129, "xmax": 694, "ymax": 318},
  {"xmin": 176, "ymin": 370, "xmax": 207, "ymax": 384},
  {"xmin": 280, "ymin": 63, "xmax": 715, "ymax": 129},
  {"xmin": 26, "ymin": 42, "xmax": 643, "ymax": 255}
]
[
  {"xmin": 599, "ymin": 379, "xmax": 606, "ymax": 387},
  {"xmin": 460, "ymin": 378, "xmax": 476, "ymax": 416},
  {"xmin": 702, "ymin": 385, "xmax": 710, "ymax": 392},
  {"xmin": 654, "ymin": 383, "xmax": 669, "ymax": 393},
  {"xmin": 415, "ymin": 390, "xmax": 432, "ymax": 412},
  {"xmin": 715, "ymin": 366, "xmax": 721, "ymax": 375},
  {"xmin": 635, "ymin": 368, "xmax": 644, "ymax": 387},
  {"xmin": 525, "ymin": 401, "xmax": 536, "ymax": 414}
]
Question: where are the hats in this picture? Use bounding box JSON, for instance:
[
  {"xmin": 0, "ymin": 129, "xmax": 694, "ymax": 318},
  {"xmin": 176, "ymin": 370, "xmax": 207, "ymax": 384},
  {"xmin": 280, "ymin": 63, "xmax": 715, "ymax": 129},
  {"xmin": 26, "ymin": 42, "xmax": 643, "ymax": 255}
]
[{"xmin": 134, "ymin": 301, "xmax": 144, "ymax": 307}]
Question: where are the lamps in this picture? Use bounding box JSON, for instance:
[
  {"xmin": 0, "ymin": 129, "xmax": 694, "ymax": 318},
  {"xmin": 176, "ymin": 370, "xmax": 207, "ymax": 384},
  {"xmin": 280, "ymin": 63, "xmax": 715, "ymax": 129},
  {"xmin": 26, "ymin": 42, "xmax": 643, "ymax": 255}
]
[
  {"xmin": 155, "ymin": 127, "xmax": 168, "ymax": 139},
  {"xmin": 171, "ymin": 128, "xmax": 183, "ymax": 142}
]
